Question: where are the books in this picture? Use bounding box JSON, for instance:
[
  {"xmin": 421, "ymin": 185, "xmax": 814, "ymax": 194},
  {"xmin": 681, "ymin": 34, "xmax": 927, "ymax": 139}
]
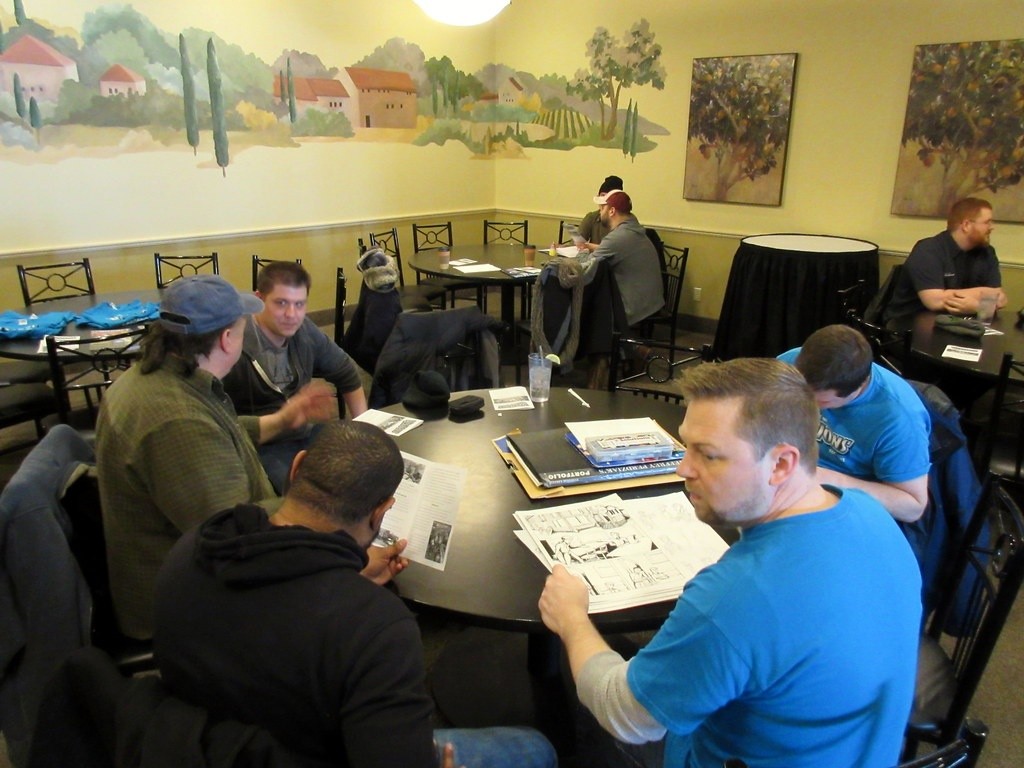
[{"xmin": 506, "ymin": 427, "xmax": 682, "ymax": 488}]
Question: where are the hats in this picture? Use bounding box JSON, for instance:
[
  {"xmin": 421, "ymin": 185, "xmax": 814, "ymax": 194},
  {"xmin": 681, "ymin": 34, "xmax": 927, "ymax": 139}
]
[
  {"xmin": 593, "ymin": 188, "xmax": 633, "ymax": 211},
  {"xmin": 598, "ymin": 176, "xmax": 624, "ymax": 196},
  {"xmin": 158, "ymin": 274, "xmax": 265, "ymax": 334}
]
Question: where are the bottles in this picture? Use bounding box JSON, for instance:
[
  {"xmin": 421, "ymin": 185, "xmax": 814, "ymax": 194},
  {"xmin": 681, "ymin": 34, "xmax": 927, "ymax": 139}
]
[{"xmin": 548, "ymin": 242, "xmax": 556, "ymax": 256}]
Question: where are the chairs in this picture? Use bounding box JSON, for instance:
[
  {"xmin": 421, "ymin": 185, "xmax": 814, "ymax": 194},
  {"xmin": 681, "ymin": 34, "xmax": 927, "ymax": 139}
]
[{"xmin": 1, "ymin": 220, "xmax": 1024, "ymax": 768}]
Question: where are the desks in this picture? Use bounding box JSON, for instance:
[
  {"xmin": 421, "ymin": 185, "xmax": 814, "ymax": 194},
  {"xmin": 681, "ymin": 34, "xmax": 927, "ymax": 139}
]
[
  {"xmin": 321, "ymin": 388, "xmax": 740, "ymax": 767},
  {"xmin": 894, "ymin": 308, "xmax": 1024, "ymax": 446},
  {"xmin": 712, "ymin": 233, "xmax": 879, "ymax": 365},
  {"xmin": 0, "ymin": 289, "xmax": 171, "ymax": 423},
  {"xmin": 408, "ymin": 243, "xmax": 594, "ymax": 369}
]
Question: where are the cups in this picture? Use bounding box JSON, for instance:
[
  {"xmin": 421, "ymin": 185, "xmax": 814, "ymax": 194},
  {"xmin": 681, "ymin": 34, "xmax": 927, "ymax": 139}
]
[
  {"xmin": 528, "ymin": 353, "xmax": 553, "ymax": 402},
  {"xmin": 524, "ymin": 245, "xmax": 536, "ymax": 267},
  {"xmin": 439, "ymin": 246, "xmax": 450, "ymax": 269},
  {"xmin": 977, "ymin": 289, "xmax": 1000, "ymax": 326}
]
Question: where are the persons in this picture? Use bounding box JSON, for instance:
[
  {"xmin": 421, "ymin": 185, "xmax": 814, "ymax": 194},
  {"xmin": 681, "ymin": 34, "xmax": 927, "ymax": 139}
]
[
  {"xmin": 879, "ymin": 198, "xmax": 1008, "ymax": 383},
  {"xmin": 96, "ymin": 276, "xmax": 338, "ymax": 675},
  {"xmin": 220, "ymin": 260, "xmax": 368, "ymax": 496},
  {"xmin": 775, "ymin": 325, "xmax": 932, "ymax": 522},
  {"xmin": 551, "ymin": 176, "xmax": 665, "ymax": 326},
  {"xmin": 152, "ymin": 419, "xmax": 557, "ymax": 768},
  {"xmin": 527, "ymin": 357, "xmax": 922, "ymax": 768}
]
